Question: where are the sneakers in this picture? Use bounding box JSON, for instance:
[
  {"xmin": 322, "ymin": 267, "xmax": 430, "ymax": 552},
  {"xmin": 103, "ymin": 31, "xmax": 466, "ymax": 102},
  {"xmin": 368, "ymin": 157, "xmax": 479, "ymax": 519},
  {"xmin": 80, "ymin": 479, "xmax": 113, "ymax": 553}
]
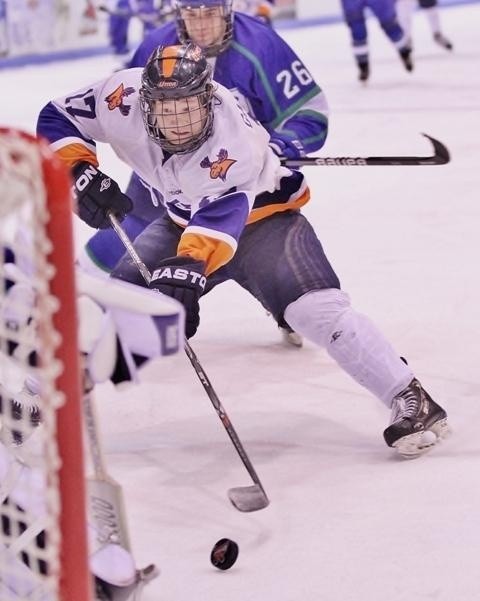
[{"xmin": 383, "ymin": 378, "xmax": 449, "ymax": 447}]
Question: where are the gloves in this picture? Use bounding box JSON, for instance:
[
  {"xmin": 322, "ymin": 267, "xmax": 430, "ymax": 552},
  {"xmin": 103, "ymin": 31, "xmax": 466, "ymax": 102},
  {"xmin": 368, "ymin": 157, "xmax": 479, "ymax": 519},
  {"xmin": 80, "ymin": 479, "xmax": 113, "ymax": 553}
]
[
  {"xmin": 64, "ymin": 162, "xmax": 133, "ymax": 230},
  {"xmin": 150, "ymin": 256, "xmax": 205, "ymax": 339}
]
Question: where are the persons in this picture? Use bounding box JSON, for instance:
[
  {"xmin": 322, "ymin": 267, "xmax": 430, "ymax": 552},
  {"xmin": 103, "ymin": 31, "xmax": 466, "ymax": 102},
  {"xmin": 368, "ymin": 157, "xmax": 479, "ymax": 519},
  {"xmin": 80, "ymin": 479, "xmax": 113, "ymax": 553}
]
[
  {"xmin": 340, "ymin": 2, "xmax": 412, "ymax": 80},
  {"xmin": 117, "ymin": 1, "xmax": 330, "ymax": 336},
  {"xmin": 398, "ymin": 1, "xmax": 453, "ymax": 51},
  {"xmin": 36, "ymin": 42, "xmax": 446, "ymax": 450}
]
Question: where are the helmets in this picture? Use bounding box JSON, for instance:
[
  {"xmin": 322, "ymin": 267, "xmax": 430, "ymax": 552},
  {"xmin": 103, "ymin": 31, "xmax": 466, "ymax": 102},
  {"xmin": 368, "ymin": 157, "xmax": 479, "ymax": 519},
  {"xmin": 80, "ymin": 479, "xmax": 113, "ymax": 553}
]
[
  {"xmin": 139, "ymin": 44, "xmax": 216, "ymax": 155},
  {"xmin": 174, "ymin": 0, "xmax": 233, "ymax": 56}
]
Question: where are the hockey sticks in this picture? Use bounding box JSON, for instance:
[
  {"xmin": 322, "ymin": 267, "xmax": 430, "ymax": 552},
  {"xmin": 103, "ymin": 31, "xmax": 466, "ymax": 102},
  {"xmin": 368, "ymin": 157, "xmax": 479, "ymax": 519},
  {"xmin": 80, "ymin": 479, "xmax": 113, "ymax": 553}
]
[
  {"xmin": 282, "ymin": 130, "xmax": 447, "ymax": 165},
  {"xmin": 100, "ymin": 203, "xmax": 269, "ymax": 513}
]
